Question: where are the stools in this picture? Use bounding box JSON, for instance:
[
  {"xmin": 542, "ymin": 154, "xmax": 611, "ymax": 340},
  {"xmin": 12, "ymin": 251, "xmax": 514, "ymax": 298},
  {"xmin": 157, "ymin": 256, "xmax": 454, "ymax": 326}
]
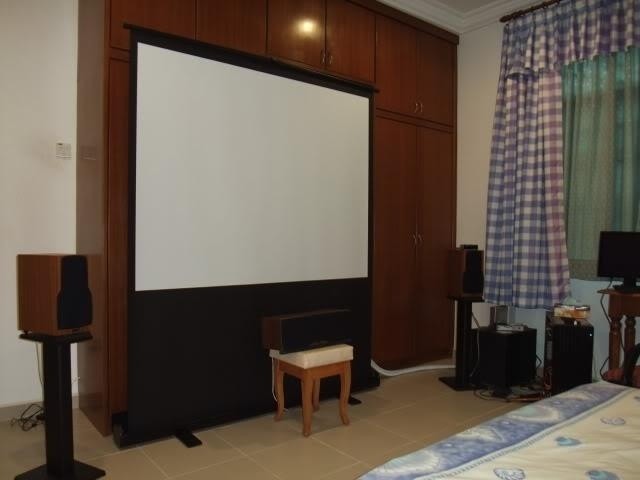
[{"xmin": 268, "ymin": 344, "xmax": 354, "ymax": 438}]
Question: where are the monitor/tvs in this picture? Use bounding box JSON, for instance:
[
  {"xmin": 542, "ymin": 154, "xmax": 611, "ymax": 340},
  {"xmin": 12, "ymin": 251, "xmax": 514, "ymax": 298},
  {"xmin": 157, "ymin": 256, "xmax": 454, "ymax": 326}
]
[{"xmin": 597, "ymin": 231, "xmax": 640, "ymax": 290}]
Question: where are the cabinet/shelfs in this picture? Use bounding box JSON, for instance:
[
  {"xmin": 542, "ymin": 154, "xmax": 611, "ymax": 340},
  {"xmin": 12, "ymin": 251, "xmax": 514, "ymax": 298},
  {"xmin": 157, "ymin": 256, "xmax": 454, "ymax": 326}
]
[
  {"xmin": 372, "ymin": 12, "xmax": 457, "ymax": 371},
  {"xmin": 108, "ymin": 0, "xmax": 375, "ymax": 84}
]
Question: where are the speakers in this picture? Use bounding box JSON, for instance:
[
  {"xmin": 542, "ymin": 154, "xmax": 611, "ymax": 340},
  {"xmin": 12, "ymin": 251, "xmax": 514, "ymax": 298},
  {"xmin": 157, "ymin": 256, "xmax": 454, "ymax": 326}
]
[
  {"xmin": 448, "ymin": 247, "xmax": 484, "ymax": 298},
  {"xmin": 17, "ymin": 254, "xmax": 93, "ymax": 337},
  {"xmin": 261, "ymin": 308, "xmax": 355, "ymax": 354}
]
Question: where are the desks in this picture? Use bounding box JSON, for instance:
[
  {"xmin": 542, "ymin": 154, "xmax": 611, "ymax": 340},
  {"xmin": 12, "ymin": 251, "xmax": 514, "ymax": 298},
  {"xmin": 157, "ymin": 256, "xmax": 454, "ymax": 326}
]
[{"xmin": 596, "ymin": 289, "xmax": 640, "ymax": 388}]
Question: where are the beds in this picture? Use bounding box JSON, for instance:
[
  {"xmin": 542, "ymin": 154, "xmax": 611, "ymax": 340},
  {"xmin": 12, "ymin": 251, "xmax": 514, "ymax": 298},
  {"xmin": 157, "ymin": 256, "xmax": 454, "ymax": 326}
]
[{"xmin": 357, "ymin": 381, "xmax": 640, "ymax": 480}]
